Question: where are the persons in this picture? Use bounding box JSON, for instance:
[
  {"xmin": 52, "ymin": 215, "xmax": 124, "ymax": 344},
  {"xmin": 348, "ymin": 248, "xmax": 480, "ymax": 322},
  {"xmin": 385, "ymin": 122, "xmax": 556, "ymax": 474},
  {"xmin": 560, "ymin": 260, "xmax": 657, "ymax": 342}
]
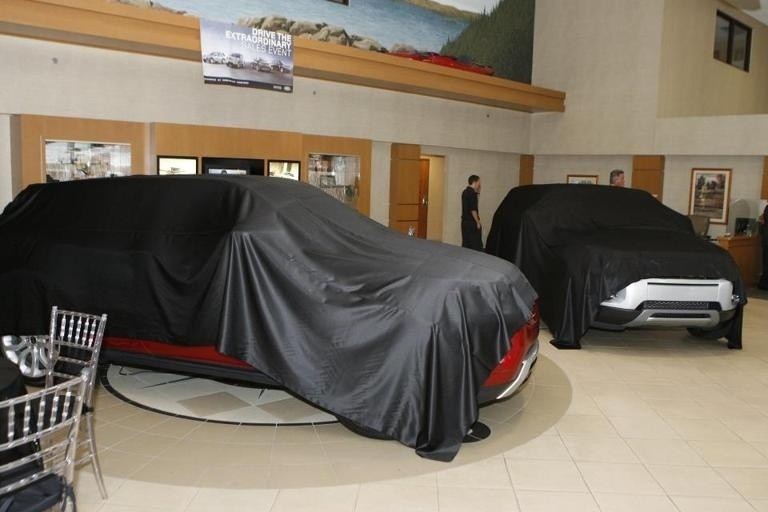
[
  {"xmin": 609, "ymin": 169, "xmax": 625, "ymax": 187},
  {"xmin": 757, "ymin": 204, "xmax": 768, "ymax": 291},
  {"xmin": 458, "ymin": 175, "xmax": 485, "ymax": 252}
]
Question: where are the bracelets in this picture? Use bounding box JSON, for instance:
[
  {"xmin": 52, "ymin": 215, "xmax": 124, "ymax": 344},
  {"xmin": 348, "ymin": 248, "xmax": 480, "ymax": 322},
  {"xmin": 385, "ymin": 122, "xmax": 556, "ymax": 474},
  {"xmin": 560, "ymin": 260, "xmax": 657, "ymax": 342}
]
[{"xmin": 476, "ymin": 192, "xmax": 480, "ymax": 195}]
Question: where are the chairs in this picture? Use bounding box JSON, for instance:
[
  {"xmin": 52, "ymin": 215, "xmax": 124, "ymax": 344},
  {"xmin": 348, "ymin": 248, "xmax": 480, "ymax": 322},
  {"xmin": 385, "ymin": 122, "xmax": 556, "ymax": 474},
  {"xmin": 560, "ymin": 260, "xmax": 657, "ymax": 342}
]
[
  {"xmin": 0, "ymin": 365, "xmax": 92, "ymax": 510},
  {"xmin": 21, "ymin": 303, "xmax": 109, "ymax": 501}
]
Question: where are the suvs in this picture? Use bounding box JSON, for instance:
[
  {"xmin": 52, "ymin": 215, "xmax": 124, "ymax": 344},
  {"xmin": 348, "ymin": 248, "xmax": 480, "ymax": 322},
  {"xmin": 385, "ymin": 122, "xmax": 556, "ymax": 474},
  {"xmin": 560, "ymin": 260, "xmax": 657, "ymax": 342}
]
[
  {"xmin": 203, "ymin": 49, "xmax": 290, "ymax": 75},
  {"xmin": 0, "ymin": 173, "xmax": 544, "ymax": 414},
  {"xmin": 484, "ymin": 179, "xmax": 744, "ymax": 336}
]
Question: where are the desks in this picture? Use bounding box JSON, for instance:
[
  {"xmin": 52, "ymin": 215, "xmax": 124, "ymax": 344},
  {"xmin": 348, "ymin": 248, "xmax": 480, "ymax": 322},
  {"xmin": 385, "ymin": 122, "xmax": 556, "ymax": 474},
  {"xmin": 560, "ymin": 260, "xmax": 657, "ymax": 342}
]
[{"xmin": 0, "ymin": 351, "xmax": 22, "ymax": 470}]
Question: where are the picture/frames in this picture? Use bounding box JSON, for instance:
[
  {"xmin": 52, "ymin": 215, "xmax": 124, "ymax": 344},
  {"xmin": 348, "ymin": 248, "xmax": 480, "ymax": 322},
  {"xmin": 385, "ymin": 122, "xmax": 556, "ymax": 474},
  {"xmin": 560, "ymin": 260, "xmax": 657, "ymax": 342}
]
[
  {"xmin": 688, "ymin": 167, "xmax": 732, "ymax": 225},
  {"xmin": 156, "ymin": 155, "xmax": 199, "ymax": 175},
  {"xmin": 567, "ymin": 174, "xmax": 598, "ymax": 185},
  {"xmin": 267, "ymin": 159, "xmax": 301, "ymax": 182}
]
